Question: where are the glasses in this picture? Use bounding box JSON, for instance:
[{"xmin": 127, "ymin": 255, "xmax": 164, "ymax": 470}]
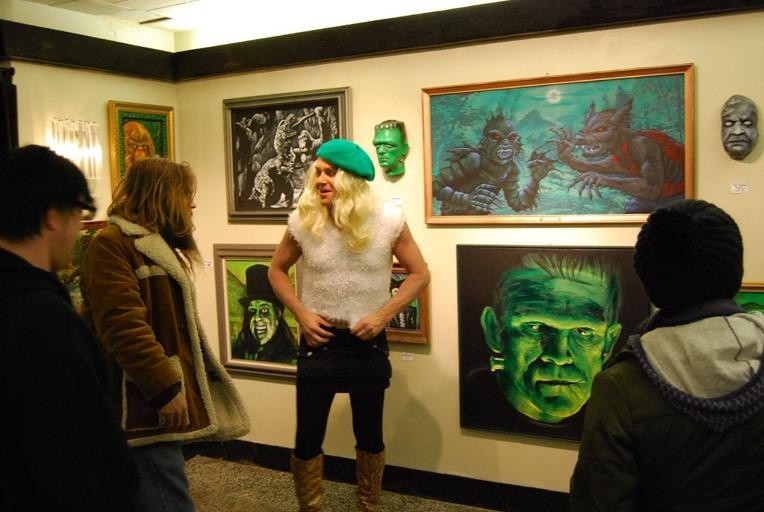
[{"xmin": 67, "ymin": 200, "xmax": 98, "ymax": 222}]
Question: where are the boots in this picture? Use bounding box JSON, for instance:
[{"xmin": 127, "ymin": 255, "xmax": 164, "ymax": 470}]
[
  {"xmin": 290, "ymin": 449, "xmax": 326, "ymax": 512},
  {"xmin": 356, "ymin": 444, "xmax": 385, "ymax": 512}
]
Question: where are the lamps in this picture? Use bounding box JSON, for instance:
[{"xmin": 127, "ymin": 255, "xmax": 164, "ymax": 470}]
[{"xmin": 47, "ymin": 118, "xmax": 101, "ymax": 180}]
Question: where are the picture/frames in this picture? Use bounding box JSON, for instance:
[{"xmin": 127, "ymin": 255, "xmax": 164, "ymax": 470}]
[
  {"xmin": 108, "ymin": 100, "xmax": 176, "ymax": 195},
  {"xmin": 732, "ymin": 281, "xmax": 764, "ymax": 313},
  {"xmin": 222, "ymin": 86, "xmax": 353, "ymax": 224},
  {"xmin": 386, "ymin": 262, "xmax": 431, "ymax": 345},
  {"xmin": 420, "ymin": 62, "xmax": 697, "ymax": 226},
  {"xmin": 456, "ymin": 243, "xmax": 655, "ymax": 443},
  {"xmin": 213, "ymin": 243, "xmax": 301, "ymax": 379},
  {"xmin": 55, "ymin": 220, "xmax": 109, "ymax": 314}
]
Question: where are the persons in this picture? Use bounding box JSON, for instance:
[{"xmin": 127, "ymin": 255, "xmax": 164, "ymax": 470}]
[
  {"xmin": 390, "ymin": 277, "xmax": 417, "ymax": 328},
  {"xmin": 721, "ymin": 95, "xmax": 759, "ymax": 160},
  {"xmin": 268, "ymin": 139, "xmax": 430, "ymax": 512},
  {"xmin": 79, "ymin": 156, "xmax": 251, "ymax": 512},
  {"xmin": 231, "ymin": 263, "xmax": 299, "ymax": 365},
  {"xmin": 460, "ymin": 250, "xmax": 623, "ymax": 441},
  {"xmin": 234, "ymin": 106, "xmax": 339, "ymax": 211},
  {"xmin": 570, "ymin": 198, "xmax": 764, "ymax": 511},
  {"xmin": 0, "ymin": 144, "xmax": 139, "ymax": 511},
  {"xmin": 373, "ymin": 120, "xmax": 409, "ymax": 177}
]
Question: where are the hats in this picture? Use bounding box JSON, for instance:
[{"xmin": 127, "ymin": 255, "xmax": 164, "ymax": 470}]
[
  {"xmin": 238, "ymin": 265, "xmax": 284, "ymax": 311},
  {"xmin": 633, "ymin": 199, "xmax": 743, "ymax": 309},
  {"xmin": 315, "ymin": 139, "xmax": 375, "ymax": 181}
]
